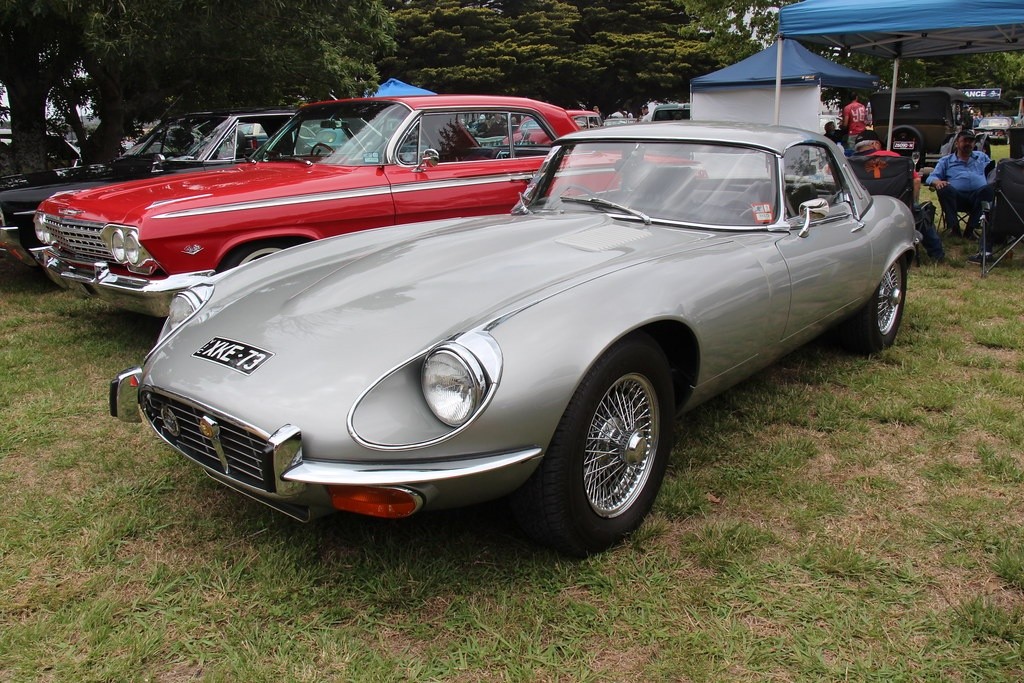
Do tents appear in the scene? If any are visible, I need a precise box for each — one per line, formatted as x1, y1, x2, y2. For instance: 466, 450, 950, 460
363, 78, 438, 99
689, 39, 880, 135
774, 0, 1024, 126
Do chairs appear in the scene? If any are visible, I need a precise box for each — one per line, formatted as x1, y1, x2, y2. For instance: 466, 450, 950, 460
846, 126, 1024, 280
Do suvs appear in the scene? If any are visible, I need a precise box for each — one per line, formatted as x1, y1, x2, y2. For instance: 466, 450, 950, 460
870, 88, 991, 172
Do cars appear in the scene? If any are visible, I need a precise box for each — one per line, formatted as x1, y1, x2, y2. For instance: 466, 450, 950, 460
109, 120, 923, 558
974, 116, 1016, 145
0, 94, 709, 318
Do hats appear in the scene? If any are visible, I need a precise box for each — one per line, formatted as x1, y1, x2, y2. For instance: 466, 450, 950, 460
855, 130, 885, 149
640, 104, 648, 109
593, 106, 598, 110
957, 130, 976, 142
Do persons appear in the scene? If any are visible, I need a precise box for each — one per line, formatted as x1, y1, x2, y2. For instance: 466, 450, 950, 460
640, 104, 652, 122
824, 122, 842, 145
973, 110, 1005, 127
593, 104, 605, 124
925, 130, 992, 241
855, 130, 950, 267
843, 91, 866, 149
967, 158, 1024, 266
473, 113, 508, 136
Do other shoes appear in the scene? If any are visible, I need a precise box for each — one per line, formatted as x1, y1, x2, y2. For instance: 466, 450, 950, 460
969, 252, 993, 262
963, 226, 980, 241
947, 228, 962, 239
